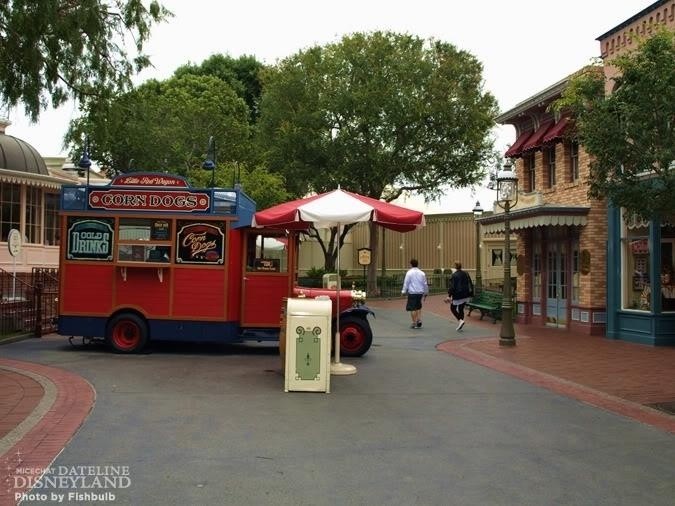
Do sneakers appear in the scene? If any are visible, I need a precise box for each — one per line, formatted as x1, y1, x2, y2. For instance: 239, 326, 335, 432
410, 321, 423, 328
456, 320, 465, 330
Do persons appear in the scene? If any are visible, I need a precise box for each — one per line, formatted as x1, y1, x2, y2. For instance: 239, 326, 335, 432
402, 258, 430, 327
444, 260, 474, 331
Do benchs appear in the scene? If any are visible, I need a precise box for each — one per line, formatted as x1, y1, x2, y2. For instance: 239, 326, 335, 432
466, 290, 504, 324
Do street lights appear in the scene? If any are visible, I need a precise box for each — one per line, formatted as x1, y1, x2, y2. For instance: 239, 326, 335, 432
471, 199, 485, 295
201, 134, 216, 213
492, 157, 521, 349
76, 133, 92, 212
230, 160, 242, 190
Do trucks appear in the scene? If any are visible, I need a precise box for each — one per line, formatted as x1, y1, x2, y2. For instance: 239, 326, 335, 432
56, 174, 376, 361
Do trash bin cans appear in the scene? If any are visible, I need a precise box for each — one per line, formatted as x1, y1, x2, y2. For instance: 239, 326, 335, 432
286, 298, 331, 394
322, 274, 341, 290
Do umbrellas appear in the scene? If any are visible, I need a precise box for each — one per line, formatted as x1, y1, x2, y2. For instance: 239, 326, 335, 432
251, 184, 427, 362
